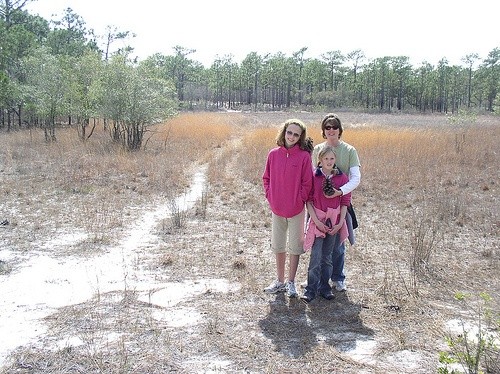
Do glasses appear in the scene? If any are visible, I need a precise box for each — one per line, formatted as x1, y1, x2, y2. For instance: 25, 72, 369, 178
325, 126, 338, 130
286, 130, 301, 137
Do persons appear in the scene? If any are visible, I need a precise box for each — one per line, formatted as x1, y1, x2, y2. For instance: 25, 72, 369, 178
303, 146, 351, 303
262, 118, 314, 298
300, 112, 361, 293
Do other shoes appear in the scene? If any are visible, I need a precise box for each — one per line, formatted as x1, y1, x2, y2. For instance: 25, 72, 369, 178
319, 287, 335, 300
300, 288, 317, 302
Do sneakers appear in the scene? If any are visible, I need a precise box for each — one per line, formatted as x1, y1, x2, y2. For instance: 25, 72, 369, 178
332, 280, 347, 292
285, 279, 298, 298
264, 278, 286, 293
300, 280, 308, 288
